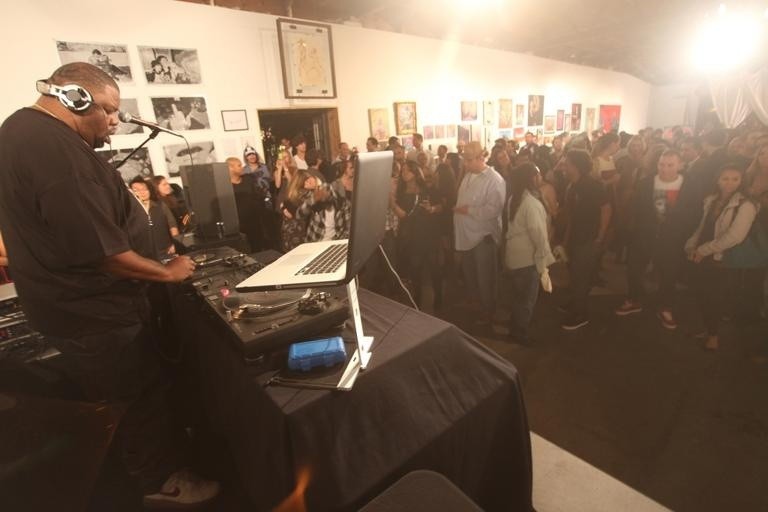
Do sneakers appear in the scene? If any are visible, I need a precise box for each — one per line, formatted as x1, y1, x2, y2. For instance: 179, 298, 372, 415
562, 313, 591, 331
143, 474, 221, 506
657, 307, 676, 329
616, 301, 643, 315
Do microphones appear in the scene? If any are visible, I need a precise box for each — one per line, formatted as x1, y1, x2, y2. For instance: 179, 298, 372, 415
119, 111, 185, 138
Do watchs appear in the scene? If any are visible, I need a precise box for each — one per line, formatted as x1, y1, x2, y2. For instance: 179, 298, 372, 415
430, 205, 434, 214
394, 204, 398, 210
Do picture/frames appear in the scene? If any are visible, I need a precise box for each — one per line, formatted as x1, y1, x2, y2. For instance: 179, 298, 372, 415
368, 107, 390, 143
556, 110, 564, 131
394, 102, 417, 135
276, 17, 337, 99
221, 109, 249, 131
544, 115, 556, 134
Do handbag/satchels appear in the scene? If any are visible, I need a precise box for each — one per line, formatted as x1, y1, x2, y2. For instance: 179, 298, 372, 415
722, 199, 763, 269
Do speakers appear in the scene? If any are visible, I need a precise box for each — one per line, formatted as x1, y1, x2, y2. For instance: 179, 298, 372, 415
178, 162, 241, 239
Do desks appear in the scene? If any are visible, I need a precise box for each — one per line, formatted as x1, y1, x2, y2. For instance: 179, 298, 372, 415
179, 248, 534, 512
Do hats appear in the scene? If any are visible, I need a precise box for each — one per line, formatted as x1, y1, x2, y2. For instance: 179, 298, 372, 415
244, 146, 255, 156
461, 141, 486, 158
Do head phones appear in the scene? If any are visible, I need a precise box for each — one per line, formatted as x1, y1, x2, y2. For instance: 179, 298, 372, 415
33, 79, 92, 113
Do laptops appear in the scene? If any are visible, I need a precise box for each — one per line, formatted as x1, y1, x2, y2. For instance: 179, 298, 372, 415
233, 151, 397, 292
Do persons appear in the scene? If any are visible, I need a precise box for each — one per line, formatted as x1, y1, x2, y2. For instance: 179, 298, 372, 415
366, 134, 465, 309
632, 124, 768, 181
273, 138, 354, 252
506, 162, 554, 348
242, 143, 273, 208
128, 176, 178, 258
150, 56, 178, 85
85, 49, 127, 79
560, 129, 631, 182
619, 137, 638, 264
153, 100, 209, 130
615, 149, 693, 330
685, 162, 758, 353
0, 232, 15, 284
560, 149, 611, 330
147, 177, 178, 223
1, 63, 219, 510
225, 157, 262, 251
453, 142, 506, 312
595, 134, 622, 256
488, 132, 566, 179
